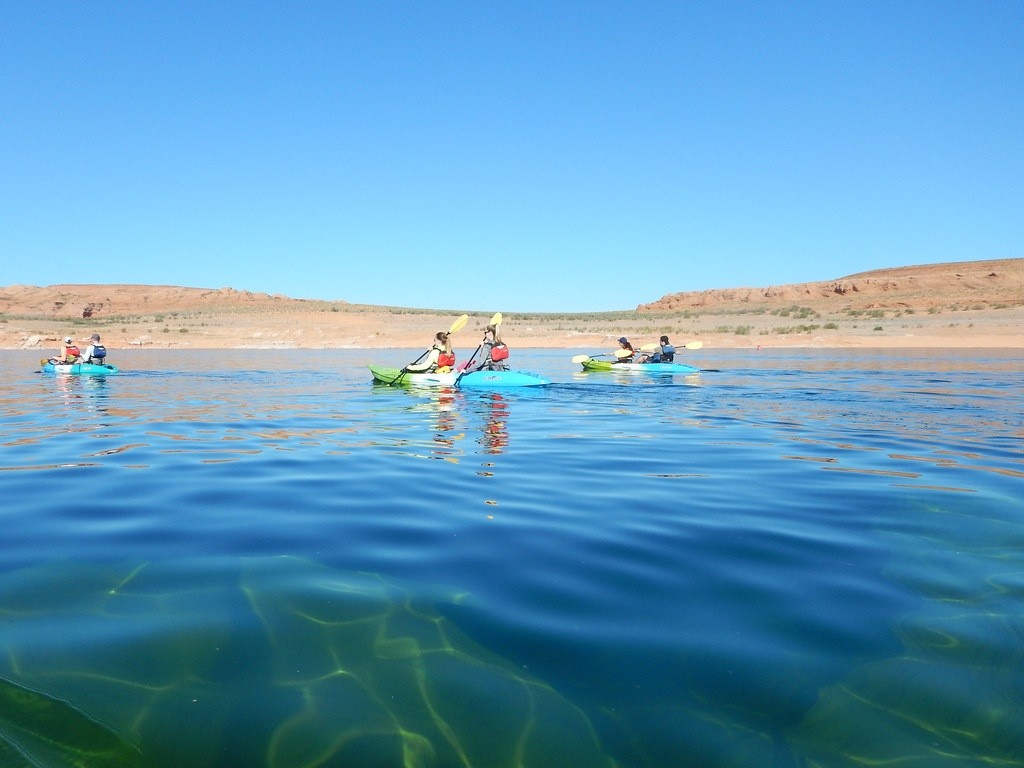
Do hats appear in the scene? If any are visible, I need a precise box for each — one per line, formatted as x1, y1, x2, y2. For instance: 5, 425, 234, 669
91, 334, 100, 339
618, 337, 627, 343
64, 336, 72, 342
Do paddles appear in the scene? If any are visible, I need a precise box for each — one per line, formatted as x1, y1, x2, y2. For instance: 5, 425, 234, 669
451, 312, 502, 386
640, 341, 704, 351
572, 349, 633, 364
40, 354, 61, 366
66, 355, 85, 363
386, 313, 469, 387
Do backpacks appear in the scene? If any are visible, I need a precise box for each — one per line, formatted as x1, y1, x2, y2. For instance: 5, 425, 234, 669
65, 346, 80, 358
433, 348, 455, 367
662, 345, 675, 353
92, 345, 106, 358
485, 342, 508, 360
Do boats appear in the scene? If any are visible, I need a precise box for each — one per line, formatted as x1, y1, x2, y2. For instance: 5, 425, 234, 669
573, 354, 701, 374
367, 364, 552, 388
40, 357, 119, 374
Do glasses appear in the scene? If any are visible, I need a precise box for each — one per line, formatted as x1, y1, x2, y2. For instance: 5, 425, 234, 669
484, 332, 488, 334
434, 339, 435, 341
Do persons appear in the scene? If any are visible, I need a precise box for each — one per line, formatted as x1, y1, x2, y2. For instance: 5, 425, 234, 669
601, 337, 634, 363
459, 325, 504, 373
52, 337, 78, 365
406, 332, 450, 374
82, 334, 106, 366
636, 336, 672, 363
